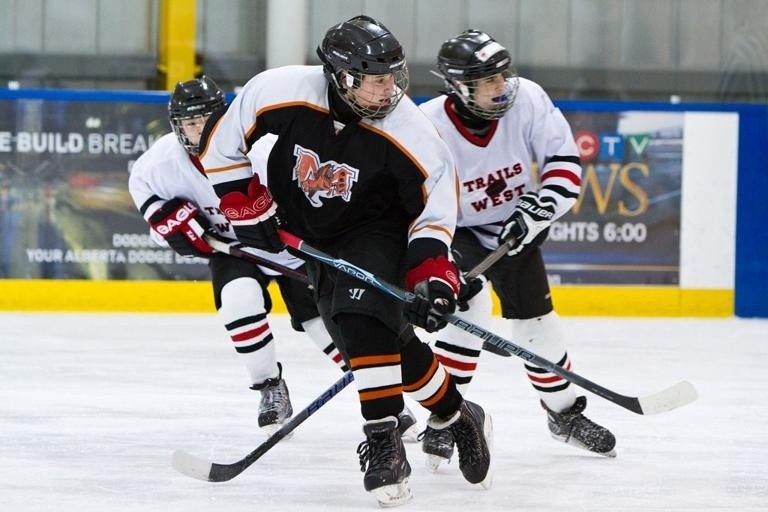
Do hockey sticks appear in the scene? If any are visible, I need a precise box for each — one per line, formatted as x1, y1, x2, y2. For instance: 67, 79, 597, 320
170, 237, 516, 482
276, 230, 707, 416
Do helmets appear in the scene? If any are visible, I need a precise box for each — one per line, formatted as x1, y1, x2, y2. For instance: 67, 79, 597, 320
315, 14, 411, 122
167, 74, 228, 155
429, 23, 521, 120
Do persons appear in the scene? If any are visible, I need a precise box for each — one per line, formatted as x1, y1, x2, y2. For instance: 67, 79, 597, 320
128, 75, 416, 438
416, 29, 616, 460
199, 14, 490, 495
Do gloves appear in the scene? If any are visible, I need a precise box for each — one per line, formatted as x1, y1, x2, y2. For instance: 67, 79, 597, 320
135, 195, 213, 258
217, 173, 290, 254
498, 189, 559, 258
400, 255, 458, 332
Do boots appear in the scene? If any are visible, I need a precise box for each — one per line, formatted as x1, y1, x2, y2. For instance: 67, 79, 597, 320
420, 399, 491, 483
356, 416, 412, 492
538, 395, 617, 452
248, 363, 292, 427
